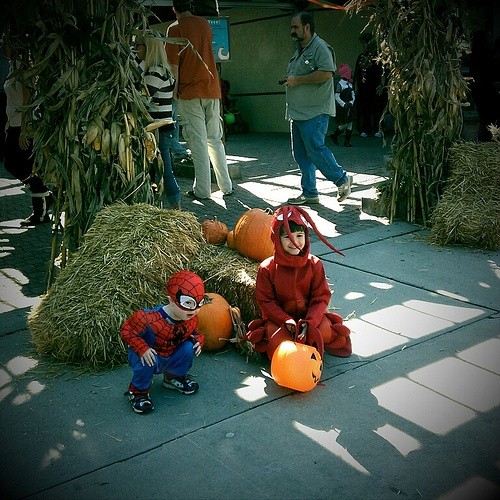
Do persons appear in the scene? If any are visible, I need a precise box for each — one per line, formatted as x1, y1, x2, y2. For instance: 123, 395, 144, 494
357, 31, 385, 138
120, 270, 205, 415
284, 10, 353, 205
255, 204, 352, 358
134, 28, 181, 209
164, 0, 236, 200
330, 63, 356, 148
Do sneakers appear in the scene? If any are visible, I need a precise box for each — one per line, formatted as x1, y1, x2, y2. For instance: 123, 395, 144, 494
374, 132, 381, 137
20, 212, 51, 226
128, 389, 154, 413
161, 372, 199, 394
337, 176, 353, 202
288, 193, 319, 204
360, 132, 368, 137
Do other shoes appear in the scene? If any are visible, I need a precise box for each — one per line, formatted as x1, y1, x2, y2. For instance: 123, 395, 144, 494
223, 187, 234, 195
188, 191, 205, 200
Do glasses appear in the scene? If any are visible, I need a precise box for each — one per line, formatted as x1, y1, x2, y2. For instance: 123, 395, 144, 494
136, 43, 145, 48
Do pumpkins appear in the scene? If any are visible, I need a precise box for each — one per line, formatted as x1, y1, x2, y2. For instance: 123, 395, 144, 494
227, 230, 235, 249
234, 207, 276, 261
194, 293, 232, 352
200, 215, 228, 245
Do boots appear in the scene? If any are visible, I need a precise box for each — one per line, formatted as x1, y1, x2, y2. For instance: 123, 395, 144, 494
330, 126, 344, 145
344, 127, 353, 147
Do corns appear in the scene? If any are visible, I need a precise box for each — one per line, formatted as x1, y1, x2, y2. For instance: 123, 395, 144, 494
146, 134, 155, 161
94, 134, 100, 150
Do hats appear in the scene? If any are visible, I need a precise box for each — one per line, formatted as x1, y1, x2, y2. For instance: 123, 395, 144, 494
338, 63, 353, 82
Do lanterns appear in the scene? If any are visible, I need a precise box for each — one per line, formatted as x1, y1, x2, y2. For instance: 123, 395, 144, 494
270, 339, 324, 393
